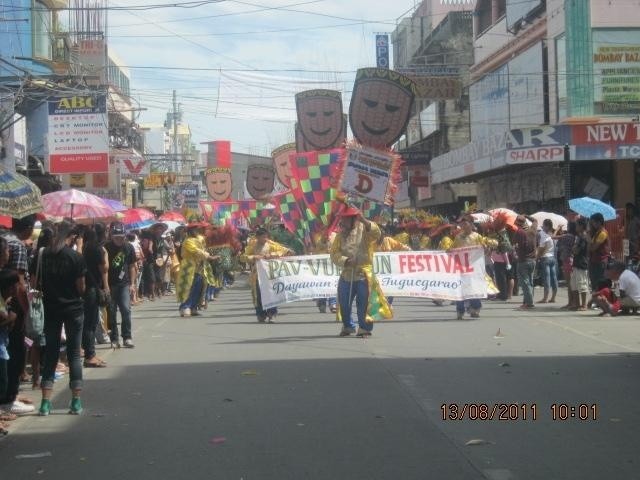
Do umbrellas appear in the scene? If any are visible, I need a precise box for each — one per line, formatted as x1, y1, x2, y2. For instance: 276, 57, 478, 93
568, 196, 617, 221
0, 164, 187, 233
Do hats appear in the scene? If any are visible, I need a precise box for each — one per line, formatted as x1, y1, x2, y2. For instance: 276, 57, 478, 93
342, 208, 359, 217
188, 223, 199, 229
199, 221, 209, 228
111, 221, 126, 237
515, 215, 527, 227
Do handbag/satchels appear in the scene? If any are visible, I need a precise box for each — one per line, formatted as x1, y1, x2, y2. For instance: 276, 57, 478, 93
98, 288, 111, 307
25, 292, 44, 337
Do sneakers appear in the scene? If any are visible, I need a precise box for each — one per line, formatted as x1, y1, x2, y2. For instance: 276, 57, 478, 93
84, 358, 107, 367
70, 396, 82, 414
0, 397, 35, 435
100, 333, 136, 347
40, 398, 51, 416
